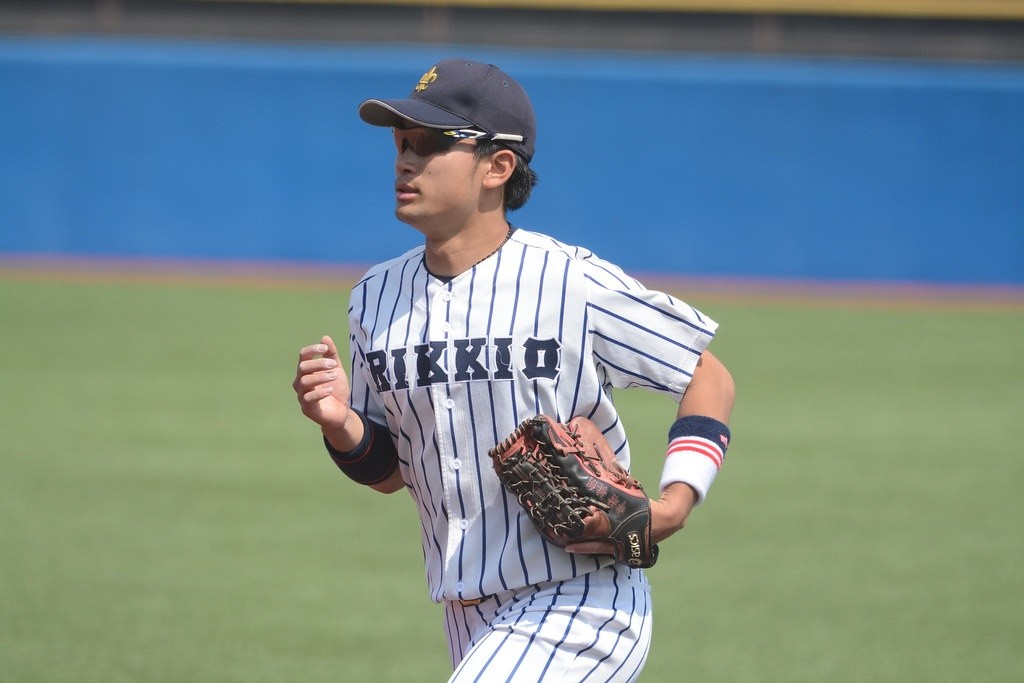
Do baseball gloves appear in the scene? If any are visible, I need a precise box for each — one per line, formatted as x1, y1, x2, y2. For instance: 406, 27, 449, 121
487, 416, 661, 571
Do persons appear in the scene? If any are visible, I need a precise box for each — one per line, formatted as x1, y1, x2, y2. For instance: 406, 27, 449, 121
293, 60, 734, 683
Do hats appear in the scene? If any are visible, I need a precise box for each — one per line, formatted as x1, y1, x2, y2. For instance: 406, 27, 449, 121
358, 58, 536, 162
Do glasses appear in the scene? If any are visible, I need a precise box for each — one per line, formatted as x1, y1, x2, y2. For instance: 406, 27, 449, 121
389, 126, 471, 156
